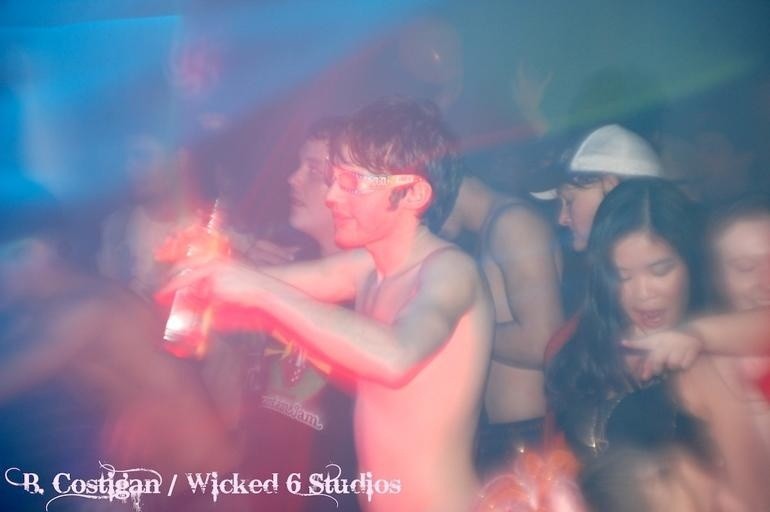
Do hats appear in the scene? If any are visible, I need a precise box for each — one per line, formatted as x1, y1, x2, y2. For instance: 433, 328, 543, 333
527, 122, 667, 202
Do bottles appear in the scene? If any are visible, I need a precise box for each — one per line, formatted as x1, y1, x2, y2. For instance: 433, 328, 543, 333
159, 196, 230, 363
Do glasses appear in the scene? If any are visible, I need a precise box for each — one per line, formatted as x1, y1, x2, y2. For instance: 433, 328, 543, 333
319, 157, 432, 200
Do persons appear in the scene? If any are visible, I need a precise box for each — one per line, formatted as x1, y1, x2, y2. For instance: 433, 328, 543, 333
1, 95, 770, 512
